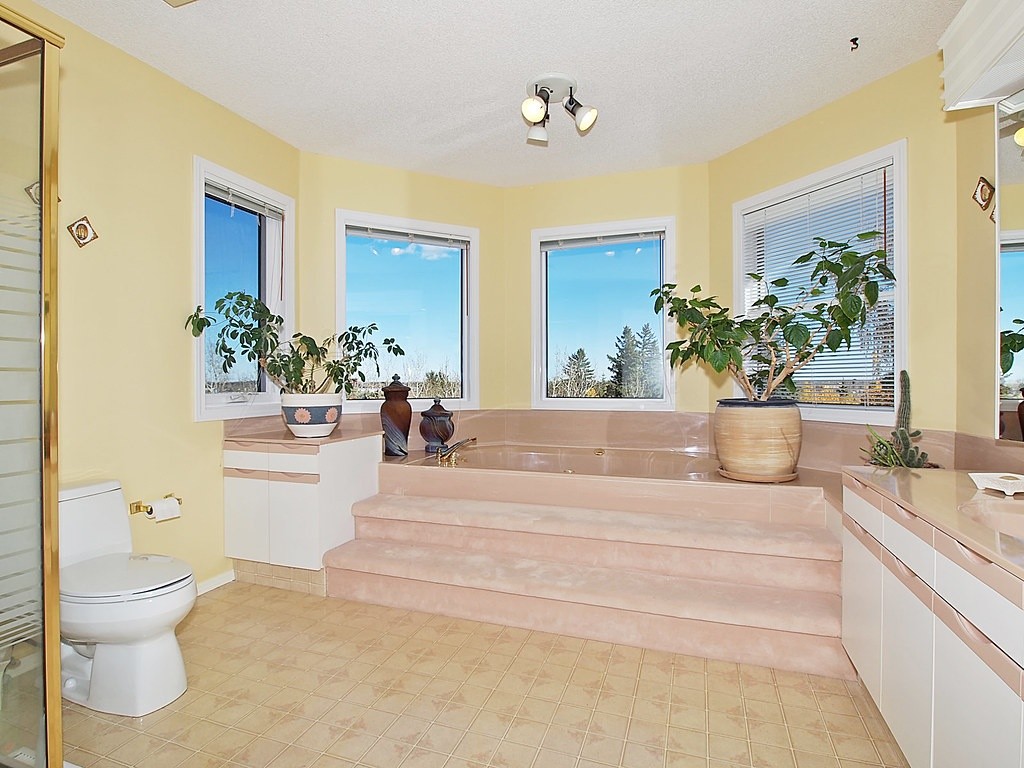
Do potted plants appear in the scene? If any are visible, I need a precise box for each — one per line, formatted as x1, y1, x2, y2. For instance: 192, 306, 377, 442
650, 230, 897, 475
184, 290, 405, 438
1000, 308, 1024, 442
859, 369, 939, 468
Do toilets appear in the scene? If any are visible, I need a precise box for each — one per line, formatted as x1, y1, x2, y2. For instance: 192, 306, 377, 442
58, 480, 198, 718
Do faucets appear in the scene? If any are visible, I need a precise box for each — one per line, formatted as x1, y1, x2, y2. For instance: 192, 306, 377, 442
436, 437, 477, 462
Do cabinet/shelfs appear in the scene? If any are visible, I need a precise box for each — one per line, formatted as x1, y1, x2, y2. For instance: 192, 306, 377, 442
839, 471, 1024, 768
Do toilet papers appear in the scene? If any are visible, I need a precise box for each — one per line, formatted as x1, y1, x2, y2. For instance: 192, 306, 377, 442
144, 497, 181, 523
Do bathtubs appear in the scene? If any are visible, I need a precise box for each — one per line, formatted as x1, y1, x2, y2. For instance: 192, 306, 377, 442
458, 446, 722, 480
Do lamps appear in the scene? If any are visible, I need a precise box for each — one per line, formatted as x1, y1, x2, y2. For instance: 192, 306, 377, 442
527, 112, 549, 143
522, 84, 555, 123
561, 87, 598, 132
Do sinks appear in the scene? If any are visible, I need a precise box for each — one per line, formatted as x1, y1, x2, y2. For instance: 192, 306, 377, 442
956, 496, 1024, 541
